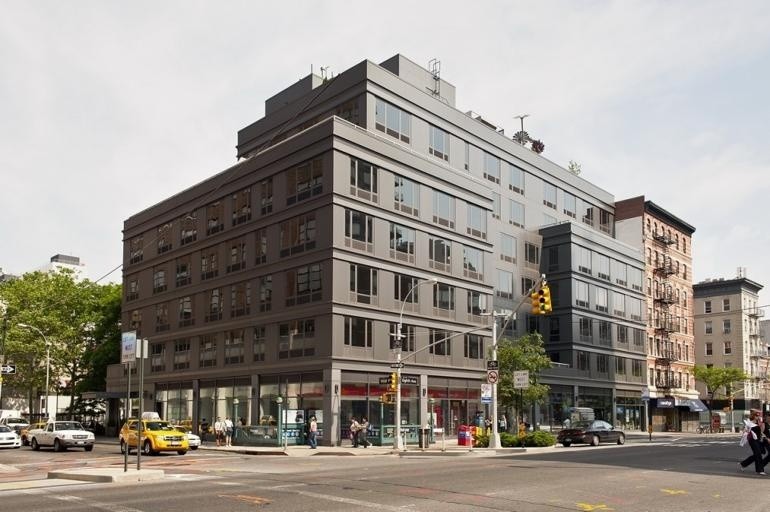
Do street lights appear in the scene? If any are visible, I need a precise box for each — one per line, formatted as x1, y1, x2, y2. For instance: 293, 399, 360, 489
233, 398, 238, 443
17, 323, 50, 421
394, 277, 438, 450
276, 396, 282, 446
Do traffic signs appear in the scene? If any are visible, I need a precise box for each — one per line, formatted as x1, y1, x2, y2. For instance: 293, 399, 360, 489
400, 377, 417, 384
391, 363, 405, 367
122, 331, 137, 361
514, 370, 530, 387
379, 378, 392, 384
1, 366, 16, 374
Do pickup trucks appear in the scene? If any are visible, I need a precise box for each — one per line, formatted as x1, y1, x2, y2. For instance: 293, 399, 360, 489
28, 420, 95, 452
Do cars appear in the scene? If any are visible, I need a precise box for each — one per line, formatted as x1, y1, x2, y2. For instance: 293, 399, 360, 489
173, 425, 201, 450
178, 419, 215, 435
21, 423, 47, 446
0, 417, 30, 429
0, 424, 21, 449
556, 419, 625, 447
259, 414, 277, 426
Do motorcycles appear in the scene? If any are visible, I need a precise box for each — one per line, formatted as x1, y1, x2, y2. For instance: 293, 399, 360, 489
695, 424, 709, 434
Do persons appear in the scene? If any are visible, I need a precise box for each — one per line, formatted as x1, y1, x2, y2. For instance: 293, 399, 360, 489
308, 417, 318, 449
199, 416, 247, 447
350, 417, 362, 447
469, 412, 530, 433
357, 416, 373, 449
761, 411, 770, 467
738, 410, 767, 476
563, 416, 571, 428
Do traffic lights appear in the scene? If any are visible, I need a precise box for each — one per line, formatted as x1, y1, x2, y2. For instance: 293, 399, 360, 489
531, 292, 540, 315
728, 397, 733, 409
538, 286, 551, 313
385, 394, 392, 403
390, 375, 395, 388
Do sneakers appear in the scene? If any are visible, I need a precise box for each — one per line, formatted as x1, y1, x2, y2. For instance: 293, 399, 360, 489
754, 470, 768, 476
736, 462, 745, 474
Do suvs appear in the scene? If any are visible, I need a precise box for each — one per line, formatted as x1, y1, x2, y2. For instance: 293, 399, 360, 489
119, 412, 189, 455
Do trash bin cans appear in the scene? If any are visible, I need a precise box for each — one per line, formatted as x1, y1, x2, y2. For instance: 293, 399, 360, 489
419, 429, 430, 448
